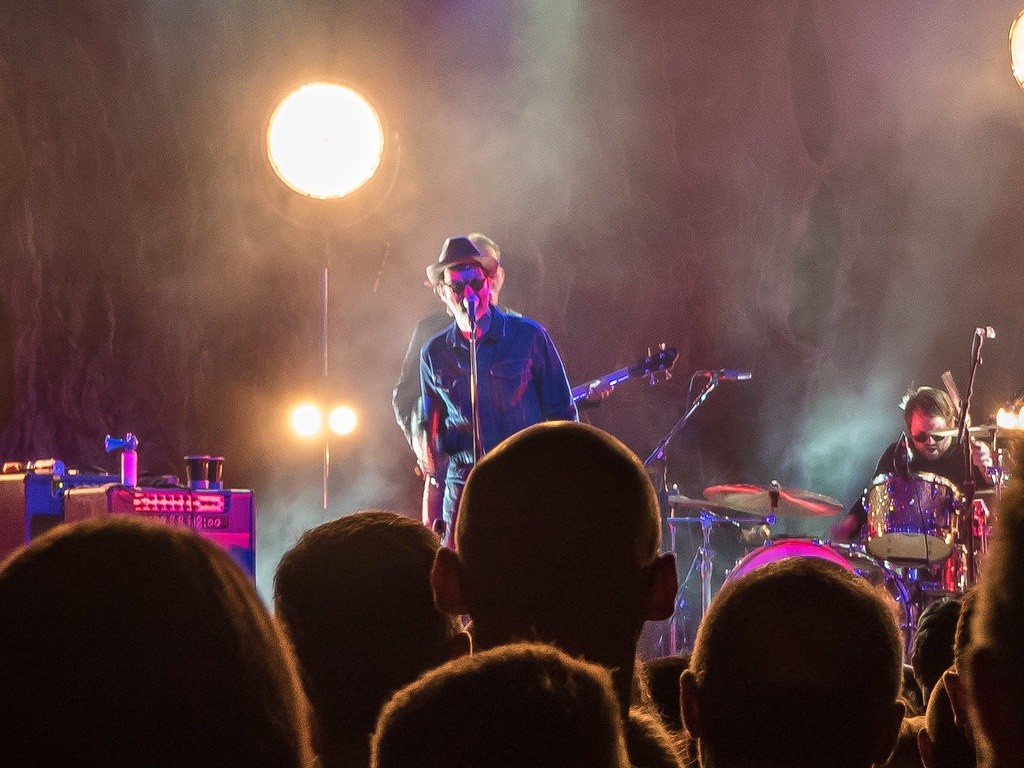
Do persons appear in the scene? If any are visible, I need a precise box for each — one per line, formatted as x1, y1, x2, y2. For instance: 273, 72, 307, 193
391, 232, 614, 549
274, 421, 697, 768
680, 435, 1024, 768
0, 518, 311, 768
833, 384, 994, 560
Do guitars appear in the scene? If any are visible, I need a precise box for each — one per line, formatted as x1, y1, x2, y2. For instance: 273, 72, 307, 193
570, 342, 680, 402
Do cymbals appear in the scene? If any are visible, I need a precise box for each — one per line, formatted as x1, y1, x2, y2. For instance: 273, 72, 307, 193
926, 425, 1001, 438
702, 483, 845, 517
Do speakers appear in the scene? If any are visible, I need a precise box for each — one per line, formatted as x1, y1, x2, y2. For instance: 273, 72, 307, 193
0, 474, 119, 564
62, 482, 256, 597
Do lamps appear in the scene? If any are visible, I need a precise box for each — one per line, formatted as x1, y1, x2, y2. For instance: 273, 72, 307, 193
248, 54, 399, 229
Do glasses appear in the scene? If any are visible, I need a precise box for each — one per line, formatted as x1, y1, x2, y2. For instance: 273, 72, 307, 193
442, 273, 489, 293
907, 429, 946, 442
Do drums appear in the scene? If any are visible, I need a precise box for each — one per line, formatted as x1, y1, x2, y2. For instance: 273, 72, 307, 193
862, 470, 962, 567
838, 547, 915, 655
884, 543, 969, 602
717, 537, 858, 591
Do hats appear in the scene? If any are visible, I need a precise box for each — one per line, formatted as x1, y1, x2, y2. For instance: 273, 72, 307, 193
425, 236, 499, 286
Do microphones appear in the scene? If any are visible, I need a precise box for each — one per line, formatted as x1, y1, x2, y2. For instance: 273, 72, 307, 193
696, 369, 752, 381
893, 432, 912, 462
975, 325, 996, 338
464, 294, 480, 323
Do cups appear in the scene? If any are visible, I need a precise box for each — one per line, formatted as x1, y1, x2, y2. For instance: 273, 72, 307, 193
208, 456, 225, 489
184, 455, 210, 490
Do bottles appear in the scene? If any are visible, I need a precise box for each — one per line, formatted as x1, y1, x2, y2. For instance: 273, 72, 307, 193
121, 433, 138, 487
